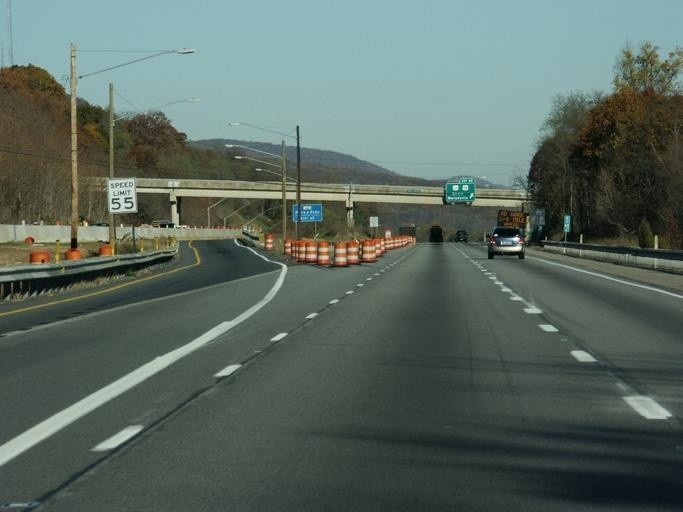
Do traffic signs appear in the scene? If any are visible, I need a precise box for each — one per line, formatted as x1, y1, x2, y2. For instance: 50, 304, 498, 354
445, 183, 475, 202
563, 215, 571, 232
459, 178, 476, 183
291, 204, 323, 222
108, 178, 137, 213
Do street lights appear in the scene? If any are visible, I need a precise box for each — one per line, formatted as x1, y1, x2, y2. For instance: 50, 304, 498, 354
66, 38, 197, 257
222, 118, 304, 254
106, 80, 204, 262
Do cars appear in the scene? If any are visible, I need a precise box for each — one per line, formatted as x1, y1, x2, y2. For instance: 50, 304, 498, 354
89, 216, 189, 229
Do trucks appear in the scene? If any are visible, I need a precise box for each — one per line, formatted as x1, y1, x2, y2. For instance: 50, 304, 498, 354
429, 226, 444, 242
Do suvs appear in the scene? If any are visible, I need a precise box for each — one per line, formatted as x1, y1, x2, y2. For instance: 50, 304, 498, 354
485, 226, 528, 259
454, 229, 469, 243
